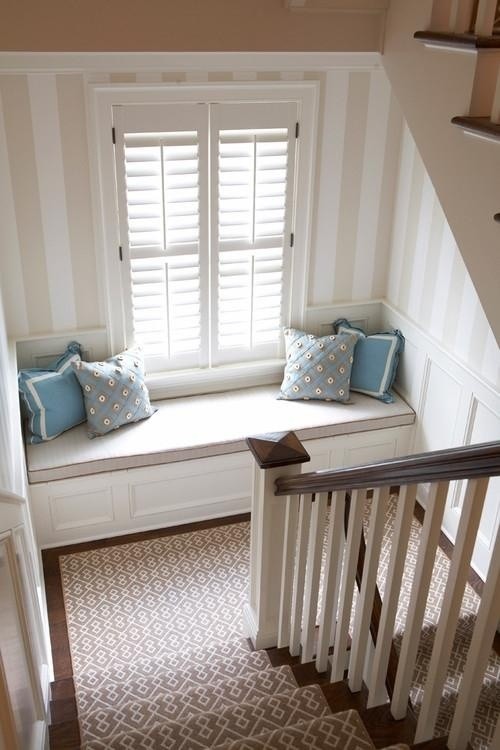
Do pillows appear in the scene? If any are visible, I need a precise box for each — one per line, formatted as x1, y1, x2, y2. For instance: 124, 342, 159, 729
276, 326, 361, 405
332, 318, 407, 405
18, 341, 91, 444
70, 346, 158, 440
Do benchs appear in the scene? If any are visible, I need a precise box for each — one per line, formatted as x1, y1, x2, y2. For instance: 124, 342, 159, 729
24, 374, 418, 550
